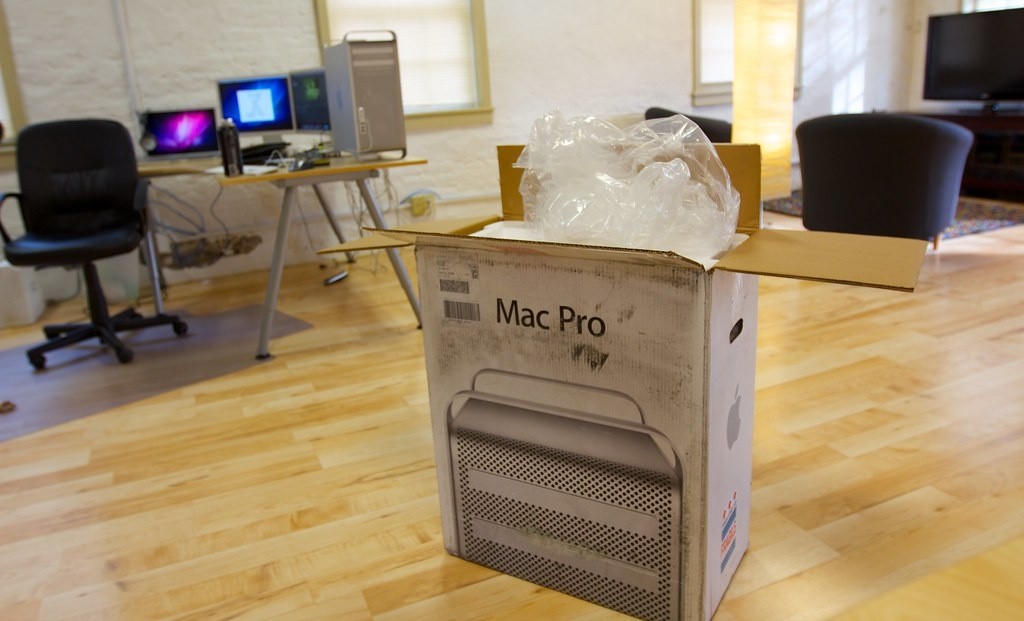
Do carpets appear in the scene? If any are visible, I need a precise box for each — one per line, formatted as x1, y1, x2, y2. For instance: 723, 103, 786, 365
760, 189, 1024, 242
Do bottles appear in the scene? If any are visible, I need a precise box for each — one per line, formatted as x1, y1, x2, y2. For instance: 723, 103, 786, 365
218, 117, 243, 178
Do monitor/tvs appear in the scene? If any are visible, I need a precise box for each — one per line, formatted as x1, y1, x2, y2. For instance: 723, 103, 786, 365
290, 70, 332, 135
143, 108, 222, 160
217, 74, 294, 143
924, 7, 1024, 117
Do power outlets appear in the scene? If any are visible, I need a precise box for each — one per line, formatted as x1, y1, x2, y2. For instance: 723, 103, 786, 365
409, 194, 436, 217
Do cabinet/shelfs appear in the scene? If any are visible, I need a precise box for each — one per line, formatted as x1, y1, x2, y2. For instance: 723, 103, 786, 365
873, 110, 1024, 202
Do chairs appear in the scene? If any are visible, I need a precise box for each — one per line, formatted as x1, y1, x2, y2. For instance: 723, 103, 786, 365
0, 119, 187, 368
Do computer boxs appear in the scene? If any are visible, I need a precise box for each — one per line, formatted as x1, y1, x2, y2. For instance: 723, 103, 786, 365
324, 30, 407, 164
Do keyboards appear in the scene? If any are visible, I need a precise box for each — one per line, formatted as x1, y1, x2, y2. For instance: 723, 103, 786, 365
204, 163, 279, 176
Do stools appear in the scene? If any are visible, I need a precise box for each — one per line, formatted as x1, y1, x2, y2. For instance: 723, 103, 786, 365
795, 113, 974, 250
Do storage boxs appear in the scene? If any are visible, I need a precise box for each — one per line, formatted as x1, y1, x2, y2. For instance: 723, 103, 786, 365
316, 145, 929, 621
0, 260, 46, 330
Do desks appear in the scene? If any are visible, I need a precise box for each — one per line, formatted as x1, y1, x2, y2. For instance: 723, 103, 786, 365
137, 149, 427, 358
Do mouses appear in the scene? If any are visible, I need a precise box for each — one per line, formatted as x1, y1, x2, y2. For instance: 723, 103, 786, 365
288, 157, 314, 172
